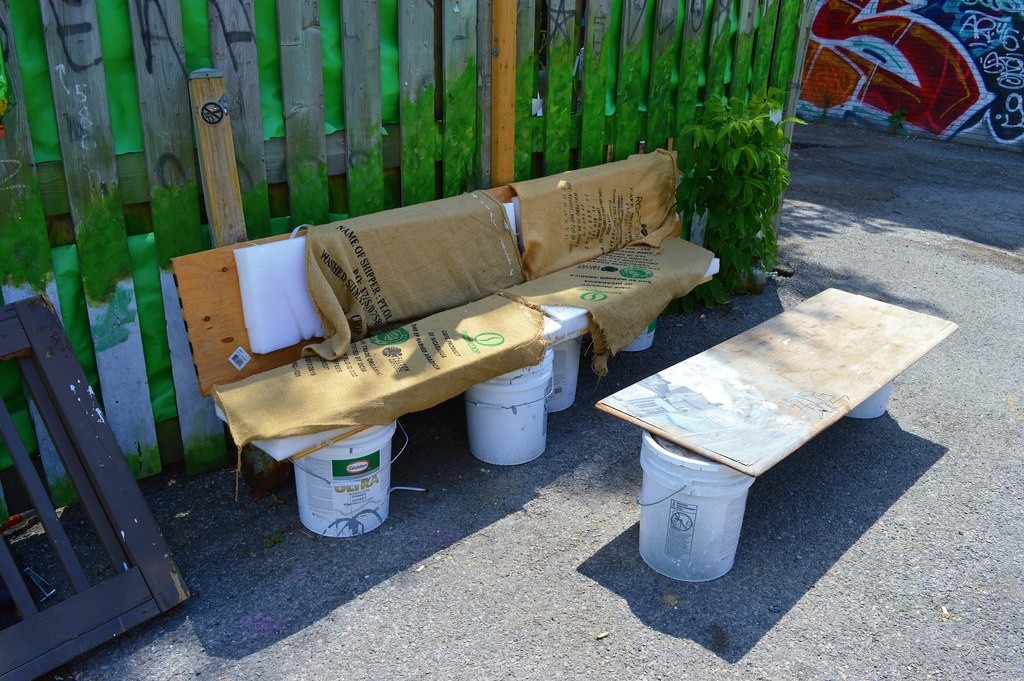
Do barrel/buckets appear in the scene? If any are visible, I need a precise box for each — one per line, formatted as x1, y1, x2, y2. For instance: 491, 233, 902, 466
619, 316, 656, 352
844, 379, 894, 419
548, 338, 582, 413
291, 420, 395, 539
465, 350, 553, 465
640, 429, 755, 582
